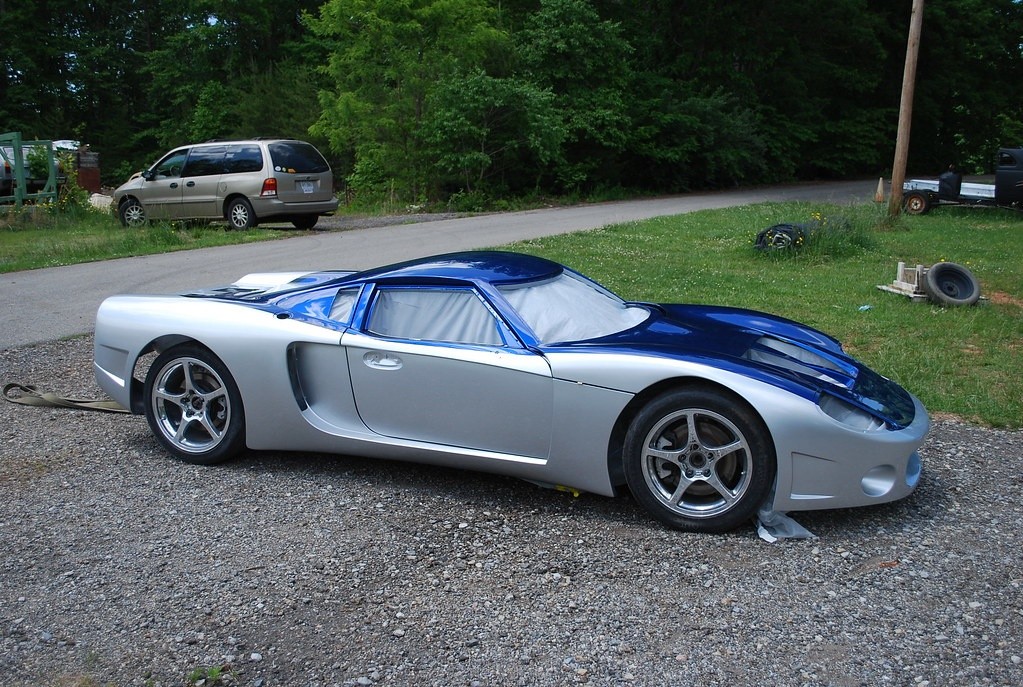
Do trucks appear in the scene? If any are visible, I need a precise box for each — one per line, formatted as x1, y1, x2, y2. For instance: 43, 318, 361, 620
900, 148, 1023, 215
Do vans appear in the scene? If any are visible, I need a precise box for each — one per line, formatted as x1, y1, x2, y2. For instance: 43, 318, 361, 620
110, 139, 341, 229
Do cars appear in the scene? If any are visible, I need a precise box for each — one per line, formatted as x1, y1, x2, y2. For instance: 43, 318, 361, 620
0, 145, 67, 204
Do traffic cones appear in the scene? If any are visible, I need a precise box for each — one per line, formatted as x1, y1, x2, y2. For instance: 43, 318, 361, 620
872, 176, 888, 204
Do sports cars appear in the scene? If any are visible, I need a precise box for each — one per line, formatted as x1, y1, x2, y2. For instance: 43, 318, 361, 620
89, 247, 934, 537
